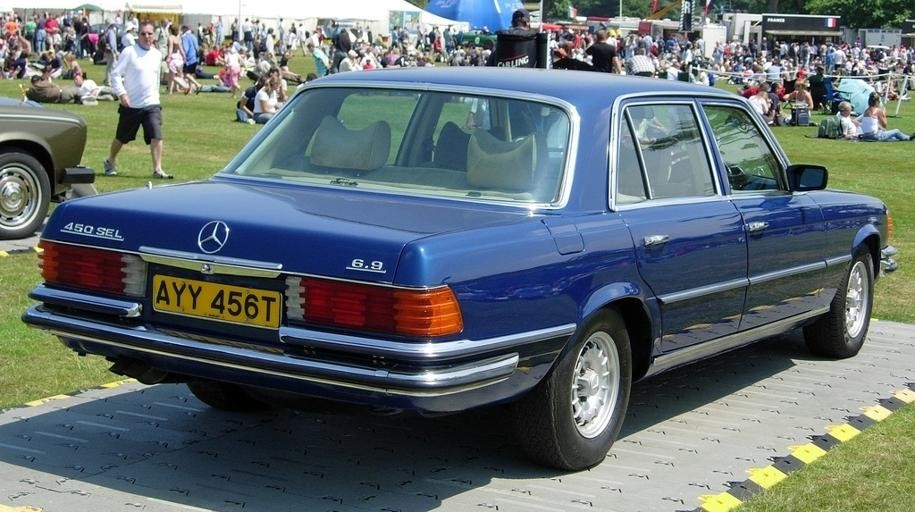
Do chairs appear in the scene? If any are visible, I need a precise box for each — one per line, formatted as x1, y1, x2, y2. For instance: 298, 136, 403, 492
820, 79, 853, 115
434, 118, 506, 172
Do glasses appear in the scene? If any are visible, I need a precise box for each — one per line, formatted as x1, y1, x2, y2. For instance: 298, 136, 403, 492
141, 31, 153, 36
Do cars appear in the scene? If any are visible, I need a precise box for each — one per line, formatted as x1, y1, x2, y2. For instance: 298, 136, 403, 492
0, 97, 94, 239
21, 66, 898, 471
89, 23, 129, 51
452, 30, 498, 51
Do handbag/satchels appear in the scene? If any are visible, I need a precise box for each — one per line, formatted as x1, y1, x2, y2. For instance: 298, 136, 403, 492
819, 116, 842, 138
792, 108, 808, 125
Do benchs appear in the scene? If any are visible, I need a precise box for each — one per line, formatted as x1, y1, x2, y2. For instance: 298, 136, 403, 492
275, 114, 559, 206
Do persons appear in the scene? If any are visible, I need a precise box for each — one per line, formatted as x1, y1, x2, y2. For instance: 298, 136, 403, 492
306, 7, 714, 128
708, 37, 914, 142
0, 7, 309, 180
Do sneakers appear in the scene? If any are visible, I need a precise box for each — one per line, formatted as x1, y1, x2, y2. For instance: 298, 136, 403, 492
104, 158, 117, 174
153, 170, 174, 179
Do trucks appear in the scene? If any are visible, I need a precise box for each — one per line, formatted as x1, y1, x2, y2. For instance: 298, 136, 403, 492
527, 11, 734, 58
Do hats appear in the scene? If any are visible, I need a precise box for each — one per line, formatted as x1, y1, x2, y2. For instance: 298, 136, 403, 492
512, 8, 530, 19
